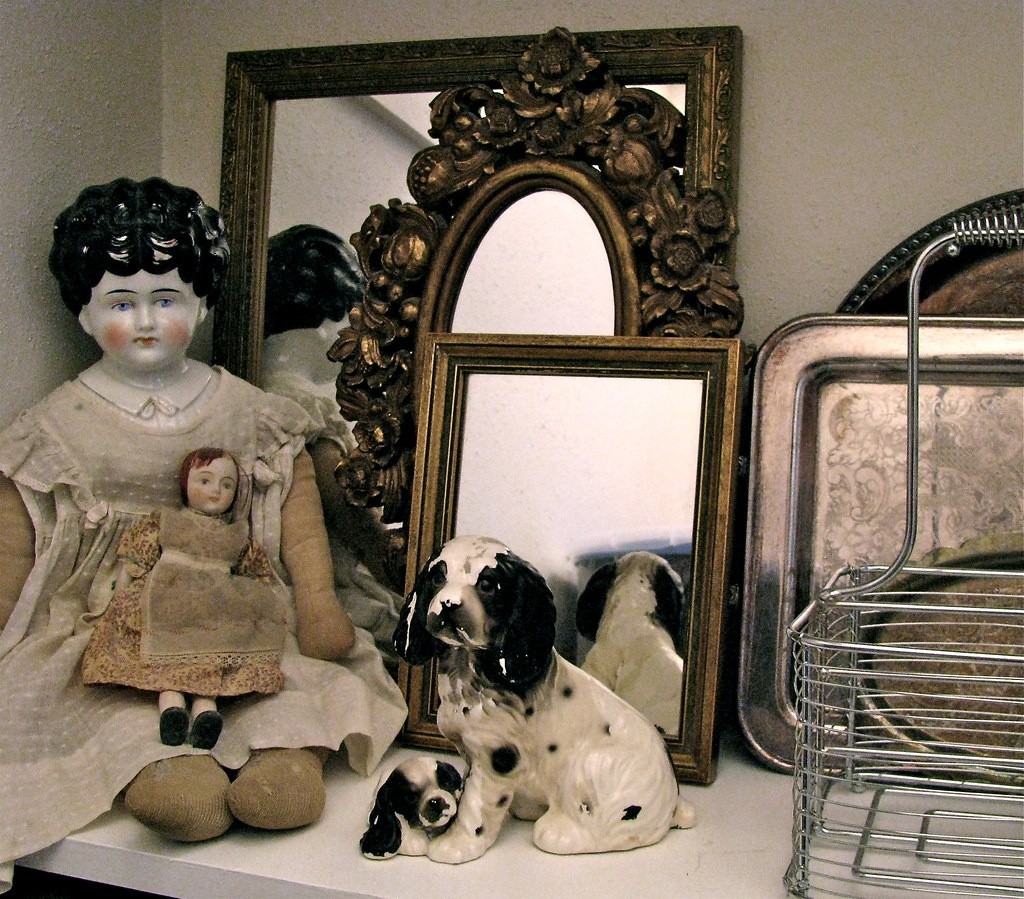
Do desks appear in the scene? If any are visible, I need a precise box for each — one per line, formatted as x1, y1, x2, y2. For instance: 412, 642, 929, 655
13, 737, 1024, 899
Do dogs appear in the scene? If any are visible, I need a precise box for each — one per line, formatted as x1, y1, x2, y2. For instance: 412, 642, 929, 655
358, 533, 697, 866
574, 550, 686, 740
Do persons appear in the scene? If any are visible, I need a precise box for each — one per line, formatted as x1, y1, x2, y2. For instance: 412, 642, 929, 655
80, 444, 290, 751
1, 178, 411, 897
263, 221, 407, 659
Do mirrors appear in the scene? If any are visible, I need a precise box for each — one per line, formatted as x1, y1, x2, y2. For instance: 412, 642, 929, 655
327, 22, 750, 605
207, 22, 747, 686
397, 330, 747, 788
